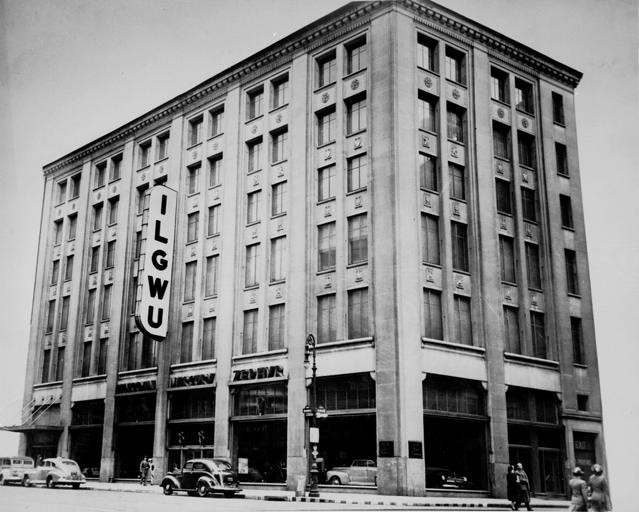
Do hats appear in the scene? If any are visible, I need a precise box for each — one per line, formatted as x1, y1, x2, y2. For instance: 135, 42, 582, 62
572, 463, 603, 475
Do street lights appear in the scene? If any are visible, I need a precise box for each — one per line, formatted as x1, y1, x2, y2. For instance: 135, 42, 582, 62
304, 334, 319, 497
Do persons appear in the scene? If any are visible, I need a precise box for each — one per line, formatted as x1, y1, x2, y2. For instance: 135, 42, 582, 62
36, 455, 45, 467
140, 456, 151, 487
589, 464, 613, 512
507, 465, 518, 510
513, 463, 534, 511
148, 458, 155, 486
566, 467, 591, 512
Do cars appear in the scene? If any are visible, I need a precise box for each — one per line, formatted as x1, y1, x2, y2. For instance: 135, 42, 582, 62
0, 455, 87, 489
426, 468, 468, 489
159, 458, 244, 497
327, 460, 377, 486
